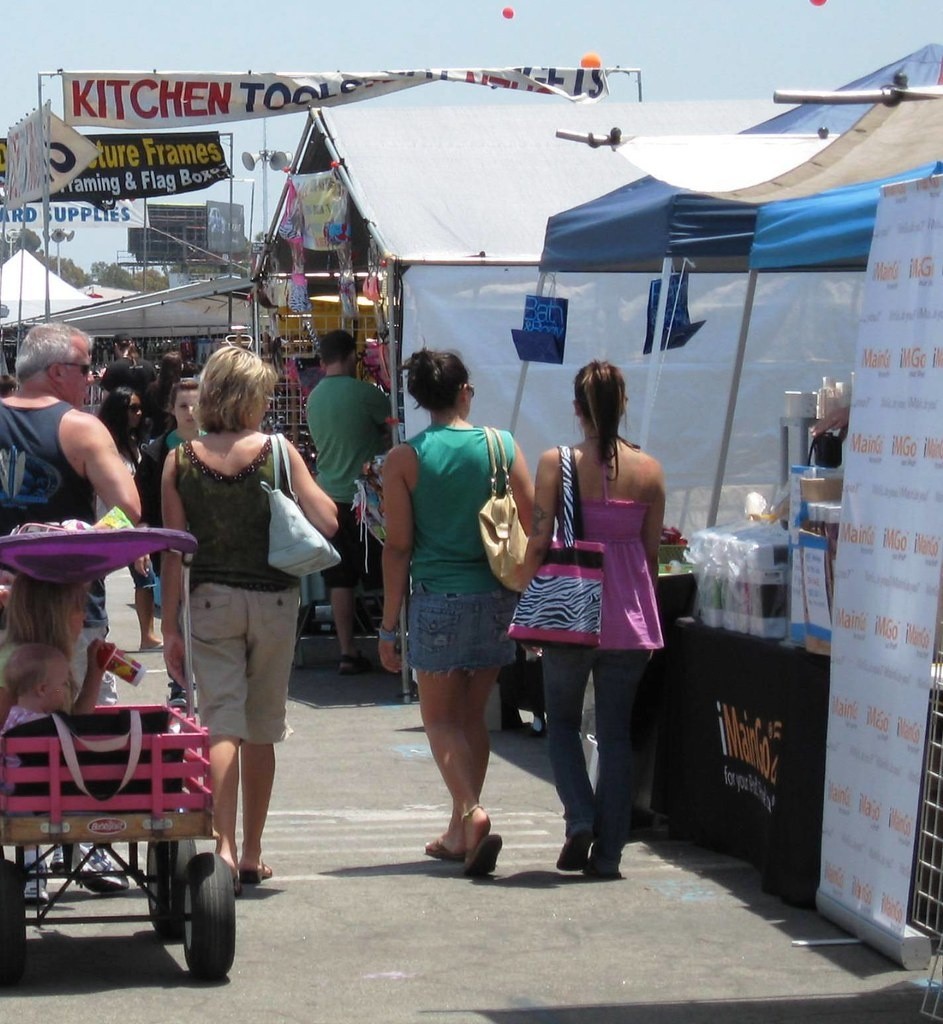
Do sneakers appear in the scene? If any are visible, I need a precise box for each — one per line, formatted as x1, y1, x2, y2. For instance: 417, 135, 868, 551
50, 842, 130, 893
23, 849, 49, 905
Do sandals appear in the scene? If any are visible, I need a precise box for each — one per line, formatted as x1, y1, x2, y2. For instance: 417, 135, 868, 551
338, 650, 372, 675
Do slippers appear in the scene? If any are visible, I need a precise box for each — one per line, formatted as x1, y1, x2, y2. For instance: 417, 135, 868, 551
139, 642, 164, 652
233, 876, 242, 897
462, 834, 502, 877
426, 837, 465, 862
239, 859, 272, 884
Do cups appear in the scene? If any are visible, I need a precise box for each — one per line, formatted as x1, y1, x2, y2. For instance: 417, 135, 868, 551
664, 563, 672, 572
785, 390, 817, 417
814, 436, 842, 467
819, 377, 848, 419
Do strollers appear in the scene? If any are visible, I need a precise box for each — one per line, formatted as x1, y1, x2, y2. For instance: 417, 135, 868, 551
1, 517, 237, 983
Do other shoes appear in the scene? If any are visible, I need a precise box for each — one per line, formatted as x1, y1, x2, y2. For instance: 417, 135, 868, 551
557, 829, 593, 871
582, 859, 622, 880
168, 680, 196, 707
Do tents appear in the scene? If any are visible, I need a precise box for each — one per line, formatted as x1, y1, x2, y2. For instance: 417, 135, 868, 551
511, 44, 942, 527
253, 99, 810, 704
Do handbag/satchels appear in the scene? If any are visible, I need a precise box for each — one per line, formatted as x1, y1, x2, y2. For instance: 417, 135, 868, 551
478, 426, 530, 593
3, 709, 184, 816
507, 445, 606, 650
643, 262, 706, 354
511, 271, 568, 364
260, 433, 342, 578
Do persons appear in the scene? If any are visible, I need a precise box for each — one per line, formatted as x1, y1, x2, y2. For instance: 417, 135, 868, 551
521, 360, 665, 876
0, 575, 107, 817
161, 346, 338, 897
377, 351, 537, 875
810, 406, 851, 436
1, 322, 141, 902
99, 333, 207, 652
306, 330, 391, 674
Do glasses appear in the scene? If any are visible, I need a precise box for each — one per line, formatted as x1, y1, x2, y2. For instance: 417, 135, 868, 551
459, 383, 475, 398
128, 404, 144, 411
46, 362, 91, 377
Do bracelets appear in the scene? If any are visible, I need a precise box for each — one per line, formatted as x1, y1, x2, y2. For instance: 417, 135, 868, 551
378, 627, 396, 640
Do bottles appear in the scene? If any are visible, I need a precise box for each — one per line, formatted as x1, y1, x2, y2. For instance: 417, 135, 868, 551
788, 464, 812, 531
97, 643, 146, 687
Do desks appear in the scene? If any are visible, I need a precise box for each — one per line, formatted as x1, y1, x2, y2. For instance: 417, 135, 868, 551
653, 612, 831, 911
